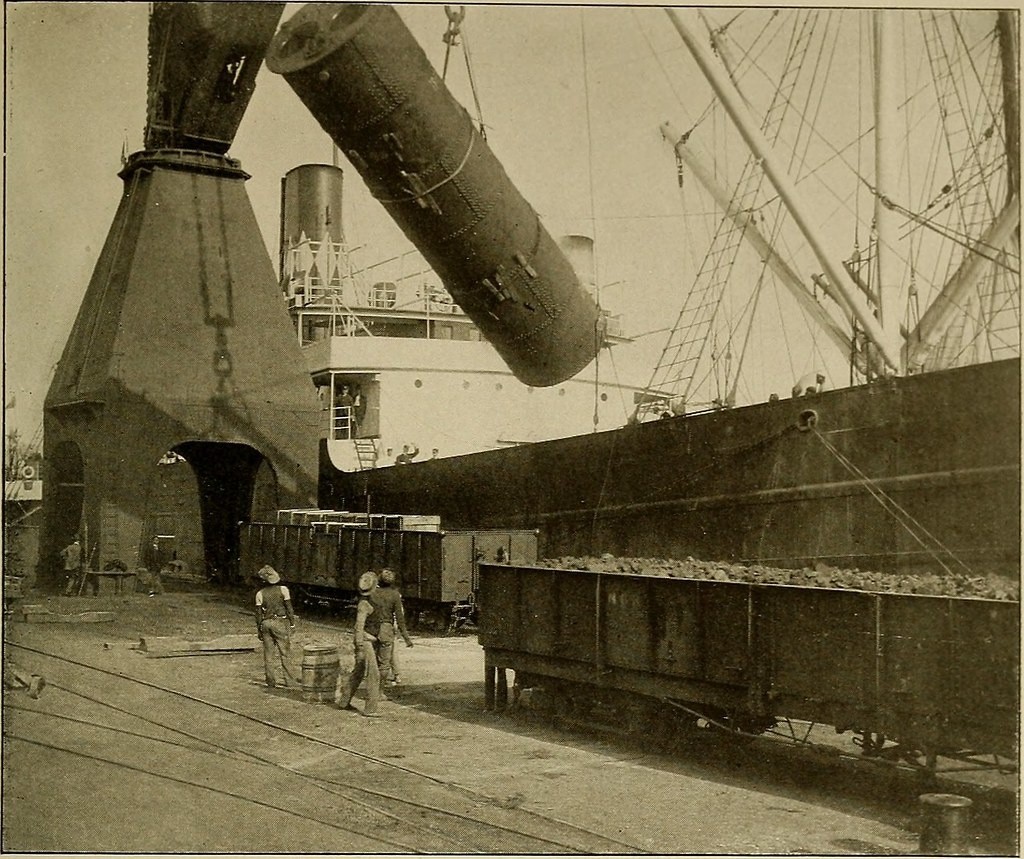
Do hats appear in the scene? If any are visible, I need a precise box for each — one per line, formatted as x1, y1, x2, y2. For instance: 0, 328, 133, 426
357, 571, 378, 596
380, 569, 395, 585
258, 565, 280, 584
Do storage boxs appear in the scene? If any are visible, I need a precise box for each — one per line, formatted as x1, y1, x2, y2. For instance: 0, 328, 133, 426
277, 508, 440, 533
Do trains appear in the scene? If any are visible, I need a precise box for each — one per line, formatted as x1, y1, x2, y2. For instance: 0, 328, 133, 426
233, 508, 539, 637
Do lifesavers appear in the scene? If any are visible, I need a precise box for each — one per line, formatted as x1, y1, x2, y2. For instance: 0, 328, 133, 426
22, 466, 35, 479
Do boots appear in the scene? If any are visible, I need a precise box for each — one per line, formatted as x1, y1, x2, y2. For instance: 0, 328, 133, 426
378, 669, 392, 702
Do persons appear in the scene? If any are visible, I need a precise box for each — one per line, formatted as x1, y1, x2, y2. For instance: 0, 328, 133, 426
59, 533, 86, 599
376, 443, 441, 468
652, 378, 819, 421
255, 562, 302, 690
144, 534, 169, 597
338, 571, 385, 721
333, 383, 367, 439
376, 568, 414, 690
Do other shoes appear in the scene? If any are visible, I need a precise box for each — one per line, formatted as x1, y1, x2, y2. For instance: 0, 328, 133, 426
362, 710, 384, 717
394, 673, 401, 683
339, 704, 357, 710
287, 678, 302, 687
